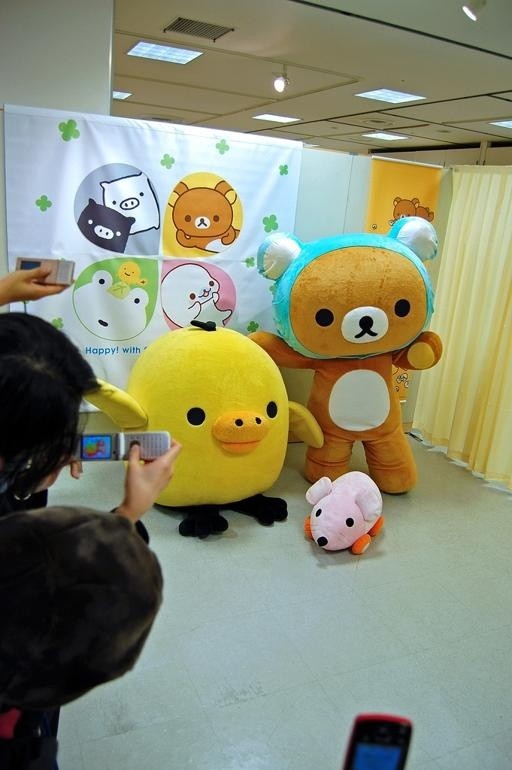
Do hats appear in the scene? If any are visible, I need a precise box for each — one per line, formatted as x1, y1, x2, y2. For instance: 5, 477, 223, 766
0, 506, 162, 705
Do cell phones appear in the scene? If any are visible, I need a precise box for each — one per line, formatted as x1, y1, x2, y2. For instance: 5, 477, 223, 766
343, 711, 412, 770
74, 430, 170, 462
14, 256, 76, 286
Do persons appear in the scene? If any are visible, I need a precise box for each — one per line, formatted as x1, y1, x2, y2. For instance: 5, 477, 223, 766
0, 265, 75, 306
0, 505, 163, 770
0, 312, 182, 543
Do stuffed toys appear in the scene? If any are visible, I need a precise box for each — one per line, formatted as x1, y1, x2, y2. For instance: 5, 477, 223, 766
305, 470, 385, 555
83, 321, 324, 538
246, 216, 443, 495
